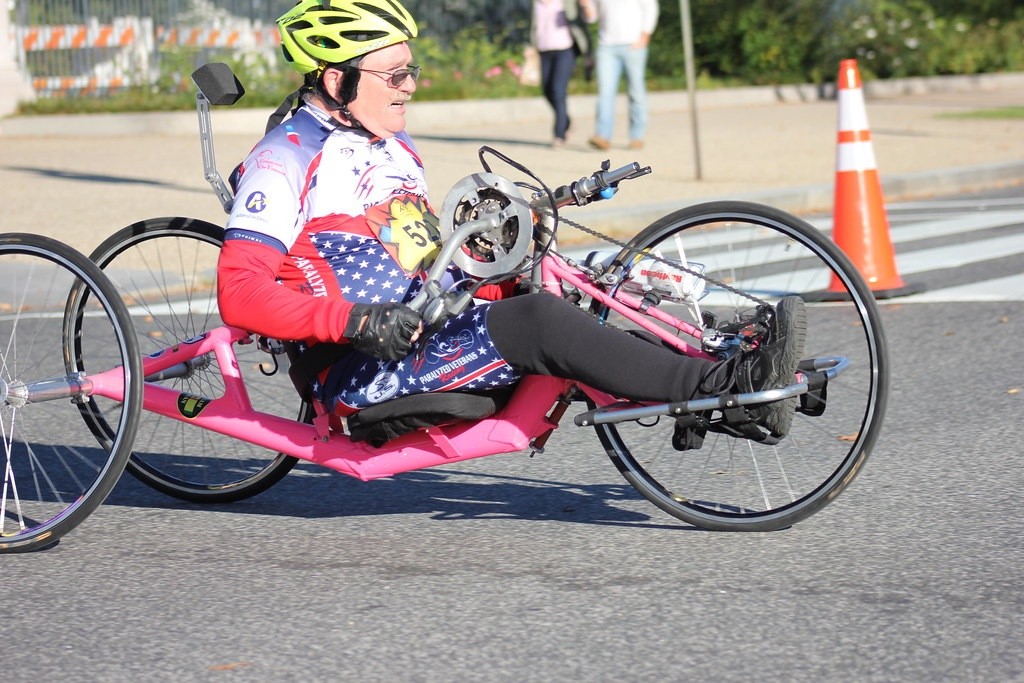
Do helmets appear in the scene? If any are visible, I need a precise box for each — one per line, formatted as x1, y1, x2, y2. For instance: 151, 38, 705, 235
276, 0, 419, 78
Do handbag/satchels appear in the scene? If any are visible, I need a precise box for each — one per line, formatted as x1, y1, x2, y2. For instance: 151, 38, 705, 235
517, 48, 543, 87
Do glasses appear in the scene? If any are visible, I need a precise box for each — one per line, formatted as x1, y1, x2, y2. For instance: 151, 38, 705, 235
359, 64, 423, 86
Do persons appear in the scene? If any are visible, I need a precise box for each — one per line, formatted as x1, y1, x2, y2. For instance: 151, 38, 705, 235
579, 0, 660, 152
528, 0, 591, 148
214, 0, 809, 445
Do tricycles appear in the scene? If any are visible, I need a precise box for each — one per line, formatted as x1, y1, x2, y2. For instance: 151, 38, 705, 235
0, 61, 889, 552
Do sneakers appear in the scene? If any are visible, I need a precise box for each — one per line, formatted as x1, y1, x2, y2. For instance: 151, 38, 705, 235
732, 295, 807, 436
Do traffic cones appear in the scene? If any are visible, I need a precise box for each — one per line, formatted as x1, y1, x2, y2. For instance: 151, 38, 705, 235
798, 58, 927, 302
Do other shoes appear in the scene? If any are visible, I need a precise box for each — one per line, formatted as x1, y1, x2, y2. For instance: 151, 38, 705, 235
588, 137, 608, 149
550, 138, 563, 149
627, 139, 644, 149
